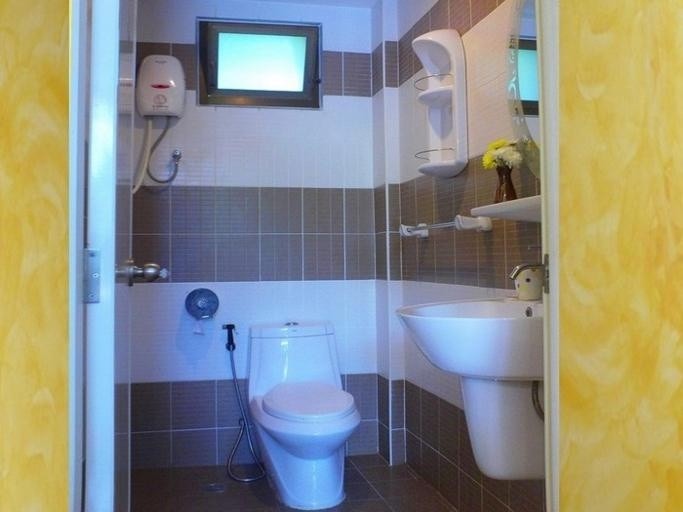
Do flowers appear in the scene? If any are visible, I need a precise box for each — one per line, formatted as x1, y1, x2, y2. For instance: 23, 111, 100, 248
480, 137, 524, 172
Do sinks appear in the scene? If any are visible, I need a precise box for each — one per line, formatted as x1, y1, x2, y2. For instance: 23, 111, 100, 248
395, 296, 544, 379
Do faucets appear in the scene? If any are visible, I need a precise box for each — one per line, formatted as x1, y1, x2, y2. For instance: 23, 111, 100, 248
509, 262, 547, 279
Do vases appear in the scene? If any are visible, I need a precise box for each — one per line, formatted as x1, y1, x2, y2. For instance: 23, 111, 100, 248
493, 165, 517, 204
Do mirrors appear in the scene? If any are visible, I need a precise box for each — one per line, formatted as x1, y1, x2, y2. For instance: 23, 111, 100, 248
503, 0, 539, 183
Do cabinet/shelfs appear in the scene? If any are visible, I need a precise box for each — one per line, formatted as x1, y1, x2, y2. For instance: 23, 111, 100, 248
410, 27, 470, 180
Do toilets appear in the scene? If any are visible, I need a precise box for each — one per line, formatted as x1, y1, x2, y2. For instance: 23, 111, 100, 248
244, 321, 362, 507
